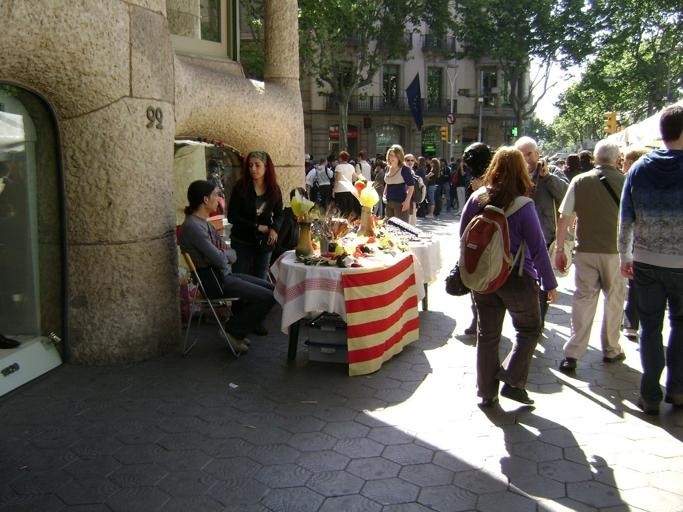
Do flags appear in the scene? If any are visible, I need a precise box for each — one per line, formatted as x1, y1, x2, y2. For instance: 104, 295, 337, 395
405, 72, 424, 131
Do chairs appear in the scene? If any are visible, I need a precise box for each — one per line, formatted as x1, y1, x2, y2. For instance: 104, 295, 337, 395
179, 243, 243, 358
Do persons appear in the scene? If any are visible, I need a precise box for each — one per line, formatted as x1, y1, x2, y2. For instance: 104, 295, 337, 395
457, 147, 559, 411
0, 333, 21, 349
0, 161, 25, 332
458, 141, 493, 337
617, 104, 683, 417
511, 135, 569, 328
382, 143, 415, 224
552, 136, 627, 376
538, 151, 653, 342
225, 150, 284, 335
175, 179, 276, 353
272, 185, 310, 265
306, 150, 473, 219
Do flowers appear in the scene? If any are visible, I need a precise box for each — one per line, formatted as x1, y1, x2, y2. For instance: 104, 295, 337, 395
291, 183, 315, 223
339, 173, 383, 207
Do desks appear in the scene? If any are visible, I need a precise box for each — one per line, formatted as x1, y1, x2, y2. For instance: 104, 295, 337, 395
277, 220, 440, 372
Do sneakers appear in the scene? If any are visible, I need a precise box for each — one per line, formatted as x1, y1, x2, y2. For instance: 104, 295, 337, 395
453, 211, 462, 216
252, 322, 268, 335
424, 215, 434, 219
482, 394, 498, 407
665, 391, 682, 406
464, 328, 475, 334
603, 349, 625, 362
501, 382, 534, 404
637, 395, 659, 415
560, 356, 576, 370
623, 327, 637, 337
218, 325, 250, 353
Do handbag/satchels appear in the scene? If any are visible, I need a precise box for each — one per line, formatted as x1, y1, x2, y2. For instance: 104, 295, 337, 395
459, 185, 535, 295
191, 265, 225, 299
412, 175, 427, 203
548, 239, 573, 277
446, 264, 471, 295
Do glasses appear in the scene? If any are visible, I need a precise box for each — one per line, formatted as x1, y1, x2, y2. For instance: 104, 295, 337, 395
407, 159, 414, 162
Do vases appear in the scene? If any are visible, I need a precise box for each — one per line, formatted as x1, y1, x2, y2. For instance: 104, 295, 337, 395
357, 206, 375, 236
